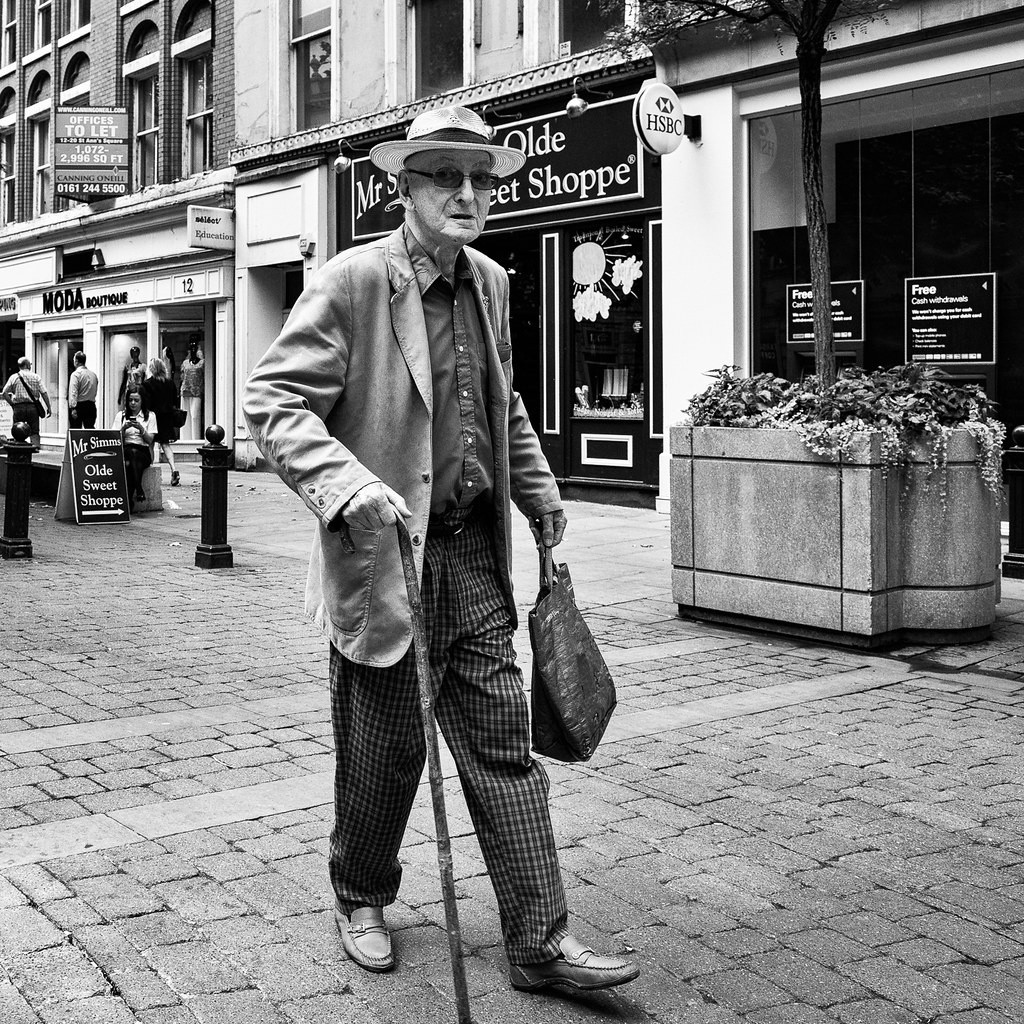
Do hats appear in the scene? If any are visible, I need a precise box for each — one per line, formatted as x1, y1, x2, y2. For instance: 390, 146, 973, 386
370, 107, 528, 176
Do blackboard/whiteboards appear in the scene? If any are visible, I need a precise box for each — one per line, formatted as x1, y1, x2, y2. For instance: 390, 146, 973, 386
54, 427, 130, 526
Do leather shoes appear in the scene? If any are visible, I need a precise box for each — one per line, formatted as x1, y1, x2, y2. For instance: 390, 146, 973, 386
335, 907, 393, 970
511, 934, 640, 990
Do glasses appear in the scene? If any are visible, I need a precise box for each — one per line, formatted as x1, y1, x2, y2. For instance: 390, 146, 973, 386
403, 168, 502, 191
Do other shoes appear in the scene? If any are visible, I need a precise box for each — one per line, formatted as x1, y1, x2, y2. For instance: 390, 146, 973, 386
171, 471, 180, 486
136, 490, 145, 502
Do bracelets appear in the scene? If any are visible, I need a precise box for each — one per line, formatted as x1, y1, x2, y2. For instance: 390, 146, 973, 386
142, 429, 145, 436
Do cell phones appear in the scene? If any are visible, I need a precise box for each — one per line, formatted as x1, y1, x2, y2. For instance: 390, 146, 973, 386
130, 417, 136, 422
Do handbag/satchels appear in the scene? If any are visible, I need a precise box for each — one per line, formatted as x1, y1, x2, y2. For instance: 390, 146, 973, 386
528, 547, 616, 762
173, 409, 187, 428
36, 400, 45, 417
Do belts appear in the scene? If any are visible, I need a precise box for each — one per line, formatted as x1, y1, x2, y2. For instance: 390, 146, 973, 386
428, 515, 477, 538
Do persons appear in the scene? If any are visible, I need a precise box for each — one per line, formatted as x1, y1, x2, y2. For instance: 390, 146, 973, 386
112, 384, 158, 514
242, 107, 640, 991
118, 347, 146, 405
180, 342, 205, 440
141, 358, 181, 486
162, 346, 175, 380
2, 357, 52, 446
69, 351, 98, 429
